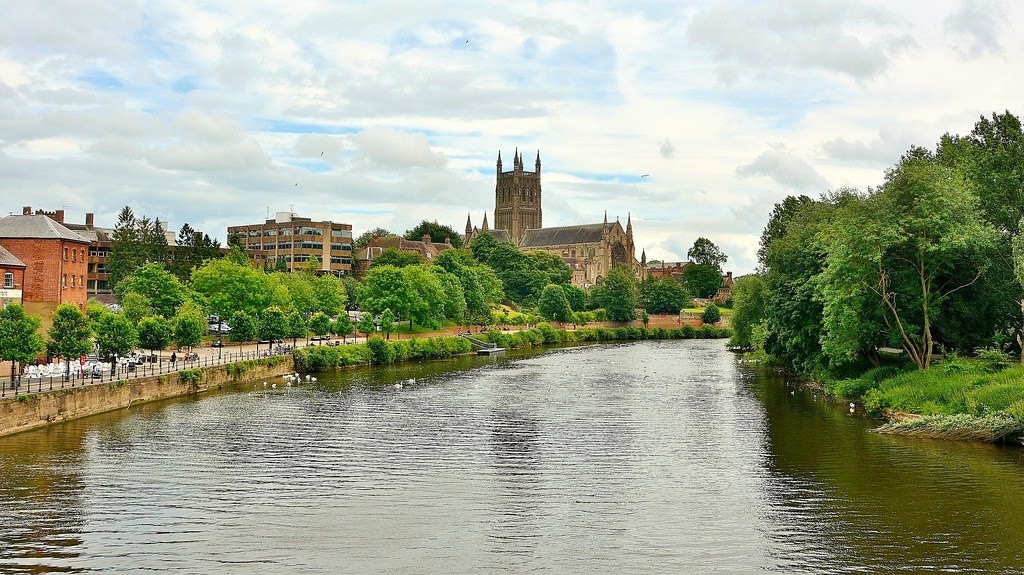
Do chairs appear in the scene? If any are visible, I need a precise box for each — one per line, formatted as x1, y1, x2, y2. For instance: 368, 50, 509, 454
23, 355, 142, 379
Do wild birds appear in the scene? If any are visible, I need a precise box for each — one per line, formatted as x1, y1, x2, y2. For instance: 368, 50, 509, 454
262, 381, 268, 387
271, 383, 277, 388
393, 377, 416, 390
849, 402, 856, 414
737, 358, 744, 364
791, 391, 794, 395
283, 371, 318, 387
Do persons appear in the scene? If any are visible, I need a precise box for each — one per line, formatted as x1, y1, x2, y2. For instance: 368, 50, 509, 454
170, 352, 176, 362
458, 326, 486, 336
185, 352, 189, 360
